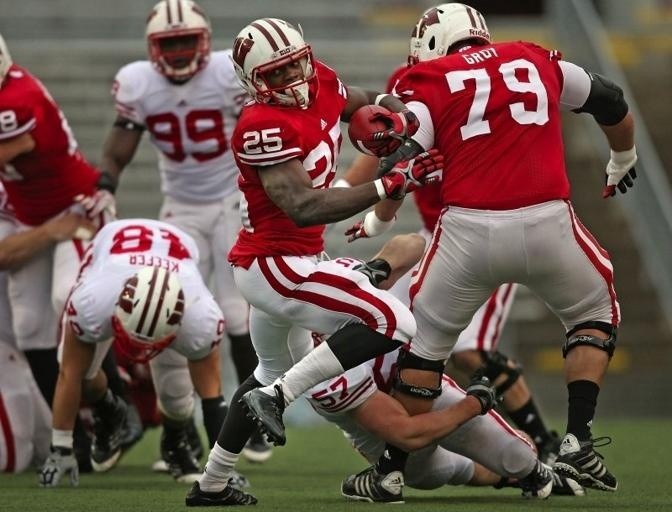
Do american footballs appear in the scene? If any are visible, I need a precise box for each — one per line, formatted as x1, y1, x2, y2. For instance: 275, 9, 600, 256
347, 105, 393, 158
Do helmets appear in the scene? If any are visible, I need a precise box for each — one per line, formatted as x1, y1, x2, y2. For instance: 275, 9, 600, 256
410, 2, 493, 60
0, 36, 12, 83
113, 266, 186, 367
145, 1, 211, 83
231, 18, 319, 110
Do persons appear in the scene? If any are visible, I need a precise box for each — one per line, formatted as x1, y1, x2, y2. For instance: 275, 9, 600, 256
1, 193, 84, 473
339, 2, 638, 505
41, 219, 230, 486
74, 1, 272, 465
331, 63, 562, 465
1, 33, 143, 475
184, 18, 444, 507
287, 230, 584, 500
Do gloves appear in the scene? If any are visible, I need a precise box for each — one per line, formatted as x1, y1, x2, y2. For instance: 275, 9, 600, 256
467, 375, 504, 417
84, 191, 117, 218
365, 107, 419, 157
230, 469, 249, 490
38, 443, 81, 487
601, 159, 638, 198
346, 219, 370, 243
378, 147, 444, 196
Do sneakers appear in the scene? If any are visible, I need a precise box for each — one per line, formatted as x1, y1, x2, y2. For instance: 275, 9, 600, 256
245, 435, 272, 462
185, 480, 257, 507
341, 468, 405, 502
518, 428, 617, 499
71, 400, 142, 474
153, 424, 204, 482
236, 385, 286, 444
